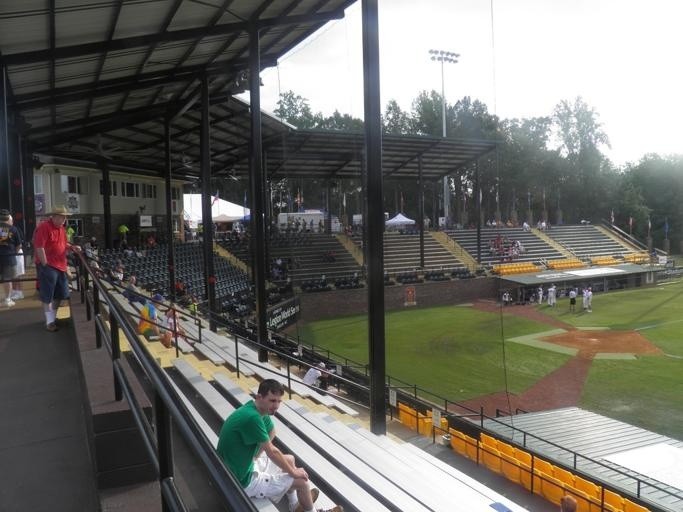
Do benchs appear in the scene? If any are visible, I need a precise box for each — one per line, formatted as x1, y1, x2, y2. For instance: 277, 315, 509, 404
88, 224, 682, 512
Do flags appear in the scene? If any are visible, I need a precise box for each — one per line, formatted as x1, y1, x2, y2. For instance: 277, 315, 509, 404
212, 192, 219, 206
610, 210, 669, 235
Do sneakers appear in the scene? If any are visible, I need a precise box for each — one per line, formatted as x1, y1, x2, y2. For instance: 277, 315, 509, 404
319, 505, 345, 512
45, 318, 60, 332
293, 488, 319, 512
5, 290, 24, 307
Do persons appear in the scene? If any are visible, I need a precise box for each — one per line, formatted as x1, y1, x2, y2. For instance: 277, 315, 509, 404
487, 231, 524, 262
397, 216, 592, 236
302, 361, 328, 396
77, 209, 363, 349
502, 283, 593, 314
558, 494, 577, 511
0, 208, 76, 309
215, 377, 344, 511
31, 203, 82, 332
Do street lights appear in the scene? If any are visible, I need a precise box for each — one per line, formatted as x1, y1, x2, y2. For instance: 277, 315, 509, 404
429, 49, 460, 229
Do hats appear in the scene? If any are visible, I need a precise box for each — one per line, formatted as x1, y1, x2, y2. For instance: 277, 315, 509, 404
45, 203, 73, 215
